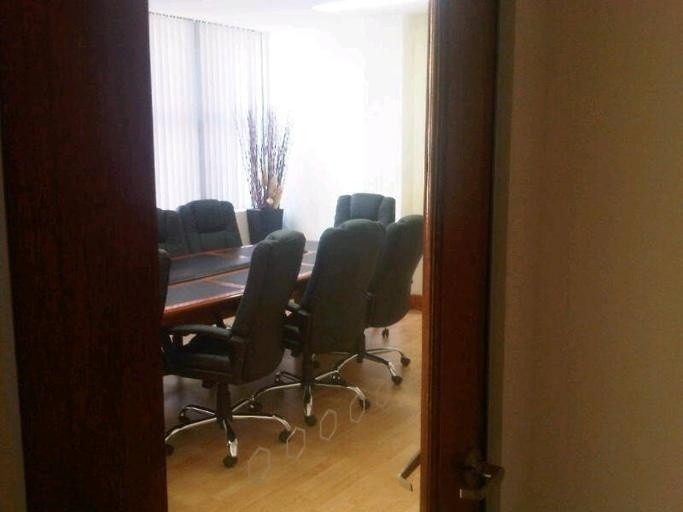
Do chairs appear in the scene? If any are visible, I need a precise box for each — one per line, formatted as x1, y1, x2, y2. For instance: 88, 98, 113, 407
324, 214, 425, 386
156, 205, 190, 255
330, 193, 399, 229
274, 218, 388, 428
162, 229, 308, 468
177, 197, 243, 251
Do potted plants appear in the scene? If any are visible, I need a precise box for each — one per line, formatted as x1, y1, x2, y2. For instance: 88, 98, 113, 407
234, 105, 294, 245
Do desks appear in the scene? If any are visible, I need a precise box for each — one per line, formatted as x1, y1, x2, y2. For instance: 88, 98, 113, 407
162, 238, 321, 335
